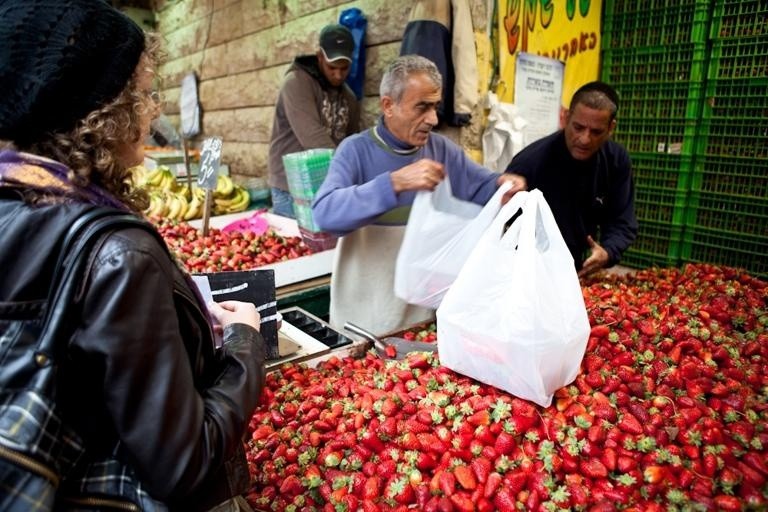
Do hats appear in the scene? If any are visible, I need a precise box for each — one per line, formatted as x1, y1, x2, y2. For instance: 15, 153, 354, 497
1, 1, 145, 140
319, 24, 355, 64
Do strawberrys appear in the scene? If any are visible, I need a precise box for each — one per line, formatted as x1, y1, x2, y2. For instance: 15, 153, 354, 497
237, 262, 767, 511
149, 216, 313, 274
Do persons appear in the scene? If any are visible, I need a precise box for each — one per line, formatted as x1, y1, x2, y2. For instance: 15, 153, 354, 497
503, 78, 641, 278
310, 50, 530, 341
266, 23, 363, 219
0, 0, 271, 512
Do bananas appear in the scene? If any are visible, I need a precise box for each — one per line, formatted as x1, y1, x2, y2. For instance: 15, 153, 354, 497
122, 166, 249, 221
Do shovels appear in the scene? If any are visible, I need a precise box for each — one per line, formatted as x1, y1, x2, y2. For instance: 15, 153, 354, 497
343, 322, 438, 362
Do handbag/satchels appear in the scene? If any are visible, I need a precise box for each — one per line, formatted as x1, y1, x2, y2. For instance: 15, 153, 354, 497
0, 300, 250, 511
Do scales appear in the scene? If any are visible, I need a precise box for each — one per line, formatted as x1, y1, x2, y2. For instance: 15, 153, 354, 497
146, 150, 199, 178
